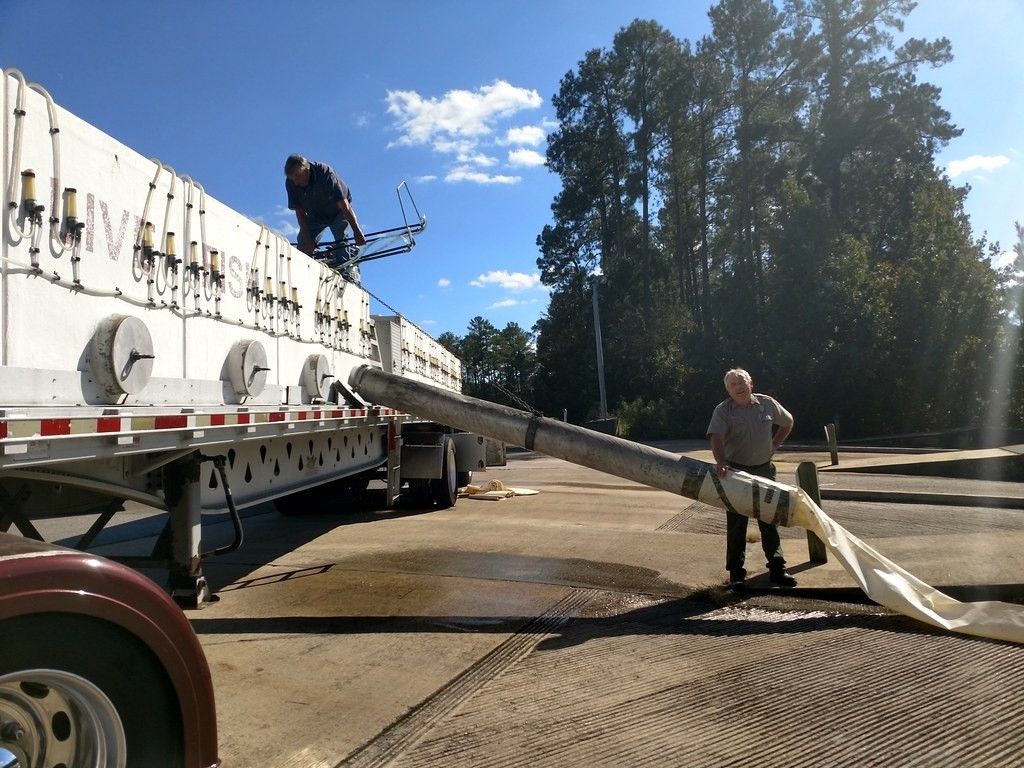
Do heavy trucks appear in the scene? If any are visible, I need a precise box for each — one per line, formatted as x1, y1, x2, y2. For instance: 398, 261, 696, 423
1, 66, 470, 613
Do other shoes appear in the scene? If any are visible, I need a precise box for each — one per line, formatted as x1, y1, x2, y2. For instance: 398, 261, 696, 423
731, 568, 747, 582
769, 572, 797, 586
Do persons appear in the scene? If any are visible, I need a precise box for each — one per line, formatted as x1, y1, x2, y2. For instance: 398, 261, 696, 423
284, 156, 367, 282
705, 368, 797, 589
